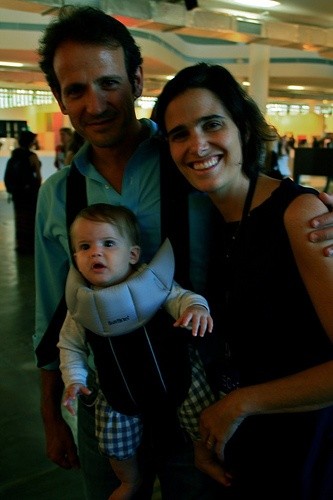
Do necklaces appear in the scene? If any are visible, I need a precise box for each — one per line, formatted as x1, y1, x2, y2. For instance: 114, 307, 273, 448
226, 176, 257, 279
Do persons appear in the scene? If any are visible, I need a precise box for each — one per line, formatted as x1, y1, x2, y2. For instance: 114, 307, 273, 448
32, 7, 333, 500
262, 129, 333, 179
56, 203, 232, 500
54, 127, 85, 170
151, 62, 333, 500
3, 131, 41, 257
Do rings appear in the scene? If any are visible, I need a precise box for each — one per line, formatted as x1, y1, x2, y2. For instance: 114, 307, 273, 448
207, 440, 214, 447
